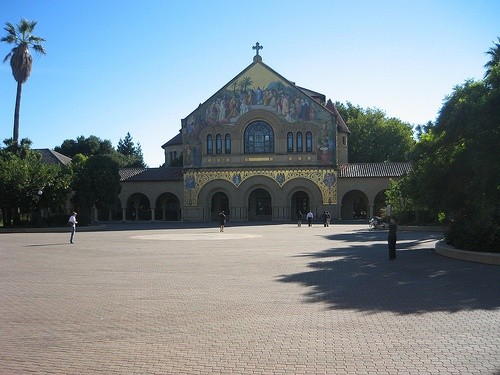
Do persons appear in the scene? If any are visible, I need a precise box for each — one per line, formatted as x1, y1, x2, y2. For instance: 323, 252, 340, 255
383, 217, 397, 260
297, 211, 303, 227
69, 212, 78, 244
307, 210, 313, 227
320, 211, 331, 227
219, 211, 226, 232
369, 218, 380, 229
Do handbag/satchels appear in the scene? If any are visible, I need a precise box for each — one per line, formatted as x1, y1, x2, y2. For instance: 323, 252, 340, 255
68, 222, 74, 226
224, 220, 227, 223
